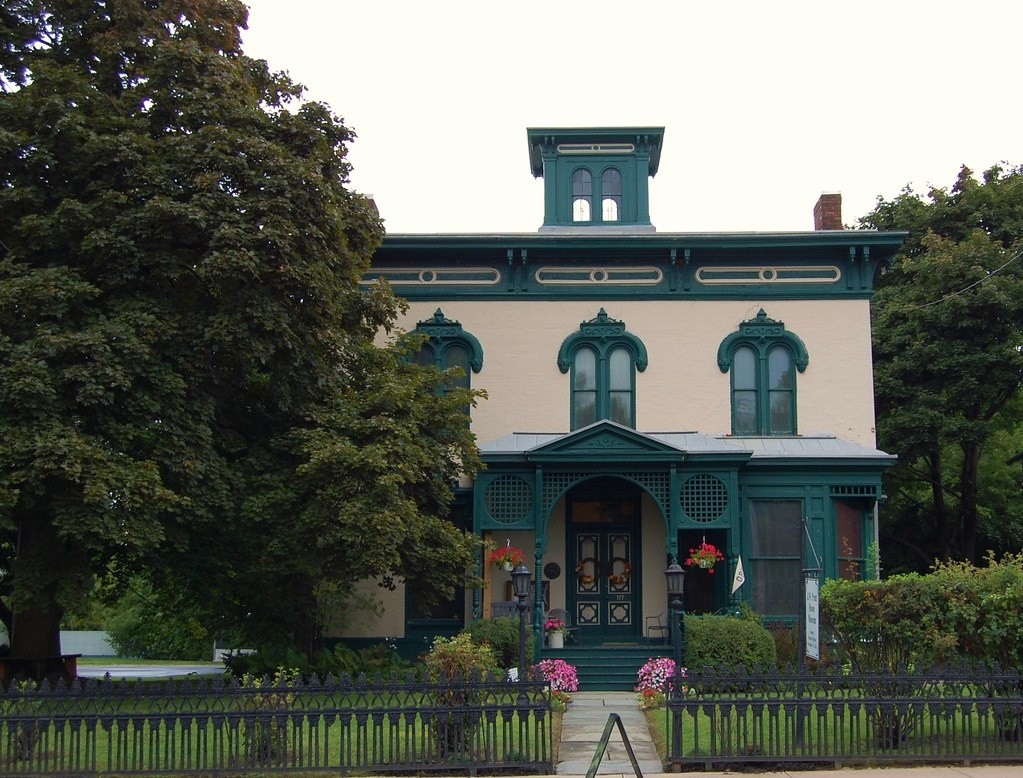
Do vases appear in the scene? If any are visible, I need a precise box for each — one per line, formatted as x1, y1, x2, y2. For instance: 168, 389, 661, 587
698, 558, 711, 568
547, 629, 564, 649
500, 560, 516, 573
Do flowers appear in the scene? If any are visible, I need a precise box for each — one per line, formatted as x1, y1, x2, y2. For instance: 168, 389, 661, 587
487, 539, 527, 571
527, 659, 580, 703
685, 535, 725, 574
610, 562, 631, 585
633, 657, 689, 710
575, 560, 595, 584
543, 617, 578, 650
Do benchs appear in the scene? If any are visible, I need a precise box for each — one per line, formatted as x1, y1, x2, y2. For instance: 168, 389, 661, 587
61, 652, 83, 677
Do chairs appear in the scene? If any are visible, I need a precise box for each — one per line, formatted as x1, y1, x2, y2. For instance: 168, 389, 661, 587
645, 608, 670, 645
